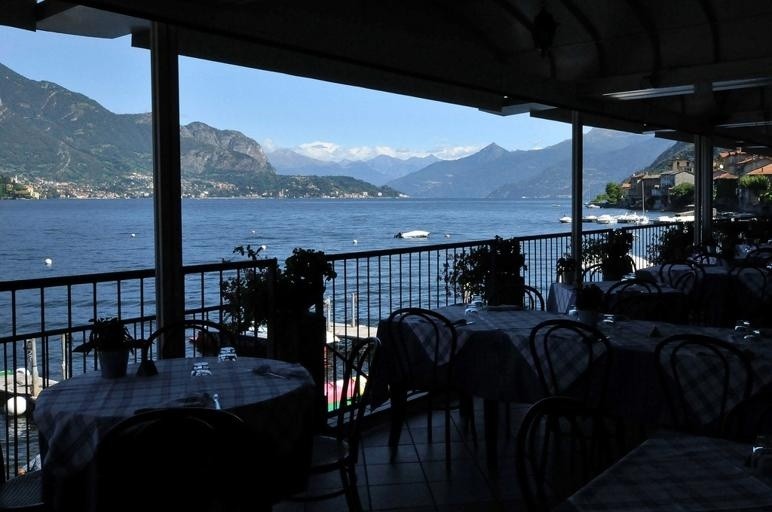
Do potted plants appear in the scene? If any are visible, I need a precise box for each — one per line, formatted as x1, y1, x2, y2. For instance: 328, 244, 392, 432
218, 246, 338, 399
73, 317, 151, 379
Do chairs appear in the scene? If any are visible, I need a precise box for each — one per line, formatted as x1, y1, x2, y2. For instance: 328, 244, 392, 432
96, 320, 379, 507
387, 215, 770, 511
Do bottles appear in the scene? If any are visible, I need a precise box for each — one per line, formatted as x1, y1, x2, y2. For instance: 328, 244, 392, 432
464, 296, 484, 325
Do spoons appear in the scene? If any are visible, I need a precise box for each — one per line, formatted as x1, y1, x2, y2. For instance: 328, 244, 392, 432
212, 393, 223, 410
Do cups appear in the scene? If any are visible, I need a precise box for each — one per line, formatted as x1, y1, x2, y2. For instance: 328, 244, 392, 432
191, 346, 238, 376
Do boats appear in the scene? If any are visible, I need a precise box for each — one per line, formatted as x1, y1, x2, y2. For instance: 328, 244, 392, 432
559, 216, 572, 223
396, 229, 431, 239
189, 317, 340, 381
582, 212, 695, 226
586, 204, 602, 210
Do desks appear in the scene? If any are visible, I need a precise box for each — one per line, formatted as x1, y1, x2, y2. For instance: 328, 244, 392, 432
39, 356, 317, 511
375, 308, 770, 465
